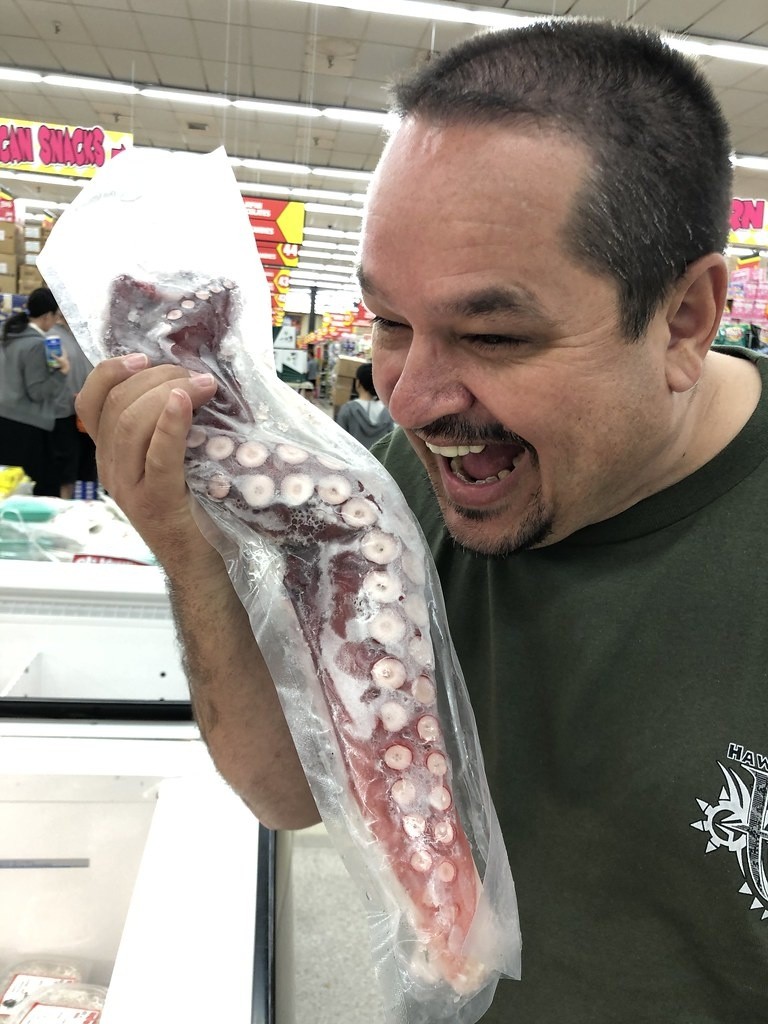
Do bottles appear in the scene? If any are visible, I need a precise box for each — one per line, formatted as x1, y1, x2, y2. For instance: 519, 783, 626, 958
72, 479, 104, 500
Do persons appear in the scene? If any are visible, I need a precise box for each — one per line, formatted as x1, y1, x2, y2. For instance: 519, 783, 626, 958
336, 363, 394, 450
75, 20, 768, 1024
0, 288, 98, 500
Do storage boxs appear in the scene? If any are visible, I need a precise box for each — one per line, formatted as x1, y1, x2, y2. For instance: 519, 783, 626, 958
331, 353, 370, 408
274, 320, 309, 382
2, 217, 61, 321
